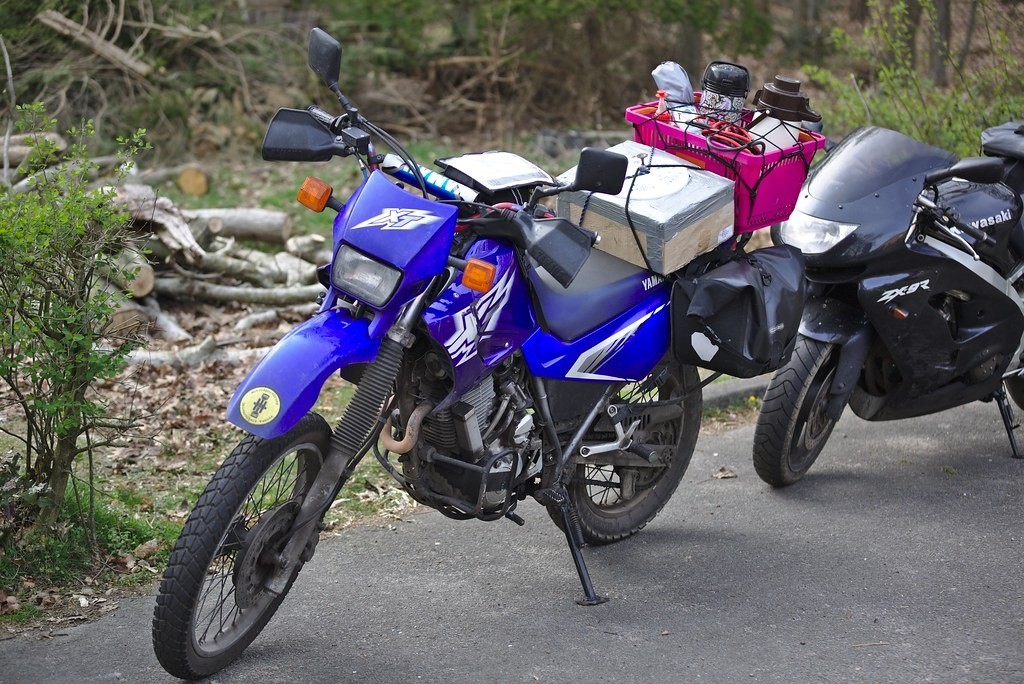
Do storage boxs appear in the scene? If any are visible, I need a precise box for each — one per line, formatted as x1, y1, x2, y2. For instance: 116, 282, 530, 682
625, 91, 826, 233
555, 139, 736, 276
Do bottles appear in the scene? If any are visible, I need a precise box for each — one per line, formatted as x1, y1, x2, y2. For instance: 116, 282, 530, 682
748, 75, 821, 155
700, 61, 749, 132
655, 91, 671, 126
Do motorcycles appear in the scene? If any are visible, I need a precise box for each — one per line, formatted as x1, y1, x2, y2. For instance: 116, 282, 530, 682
152, 26, 753, 684
755, 121, 1024, 491
